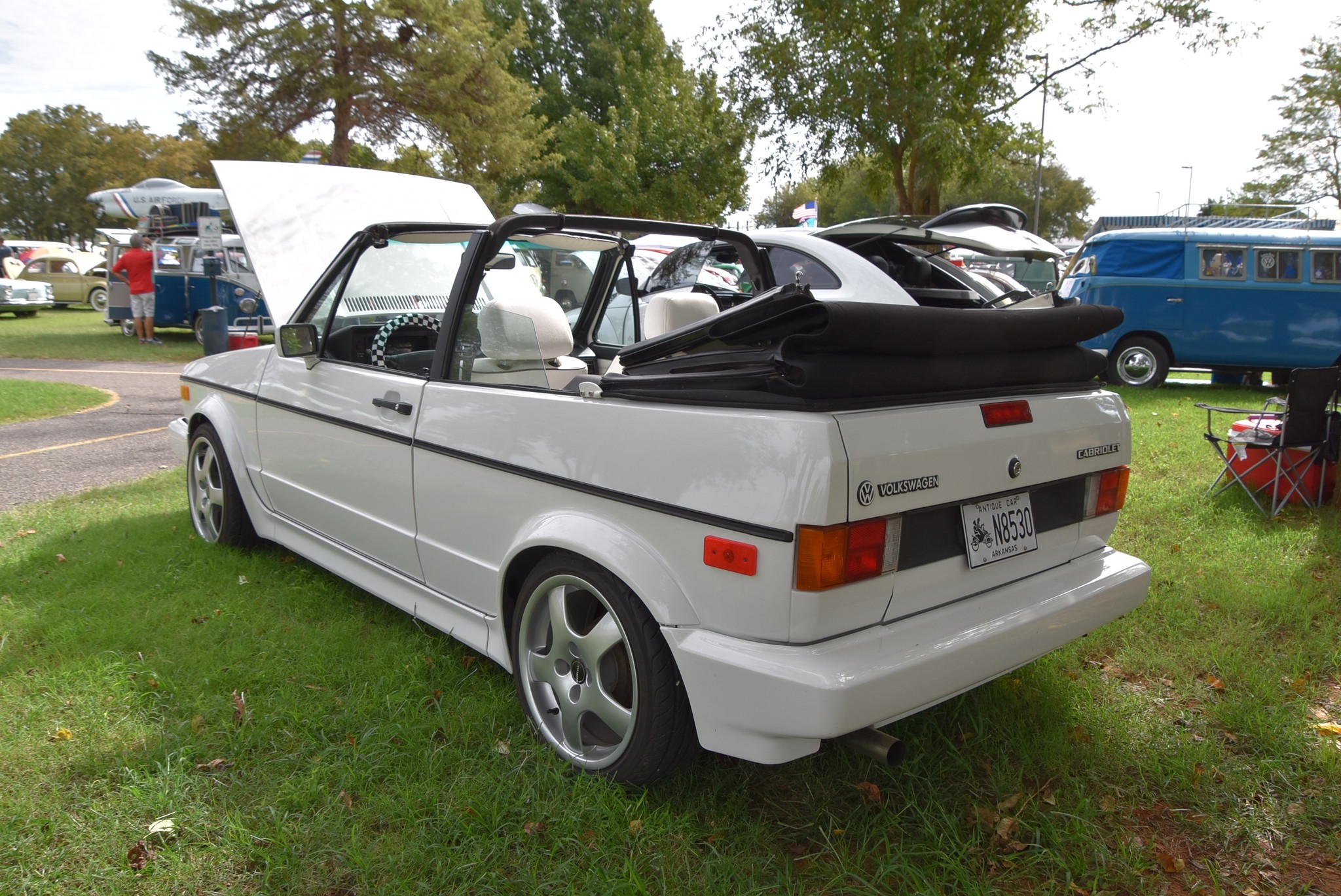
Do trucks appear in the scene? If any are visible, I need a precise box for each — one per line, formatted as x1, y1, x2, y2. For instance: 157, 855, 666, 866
528, 251, 622, 313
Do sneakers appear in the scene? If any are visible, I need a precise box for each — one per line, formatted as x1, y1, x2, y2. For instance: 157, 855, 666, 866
138, 337, 165, 345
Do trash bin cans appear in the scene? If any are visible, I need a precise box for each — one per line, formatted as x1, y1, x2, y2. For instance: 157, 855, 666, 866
199, 306, 230, 356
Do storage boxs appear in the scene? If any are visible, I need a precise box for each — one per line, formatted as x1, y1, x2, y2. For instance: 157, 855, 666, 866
143, 201, 221, 237
1226, 415, 1337, 509
228, 331, 259, 351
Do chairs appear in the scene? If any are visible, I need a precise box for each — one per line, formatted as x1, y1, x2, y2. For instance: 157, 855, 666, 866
458, 295, 589, 392
1194, 364, 1341, 522
604, 291, 720, 377
863, 254, 890, 276
901, 255, 944, 308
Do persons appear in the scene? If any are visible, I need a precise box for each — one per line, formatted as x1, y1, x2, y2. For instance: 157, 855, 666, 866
0, 237, 15, 278
111, 233, 166, 344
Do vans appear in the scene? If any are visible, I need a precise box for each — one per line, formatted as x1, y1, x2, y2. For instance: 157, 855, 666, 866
103, 233, 277, 347
1058, 204, 1341, 391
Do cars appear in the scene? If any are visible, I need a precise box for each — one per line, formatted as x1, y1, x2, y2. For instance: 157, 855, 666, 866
2, 250, 111, 313
167, 212, 1151, 785
562, 201, 1082, 346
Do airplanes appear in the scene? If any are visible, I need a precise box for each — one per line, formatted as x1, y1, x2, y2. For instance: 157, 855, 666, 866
83, 149, 323, 229
